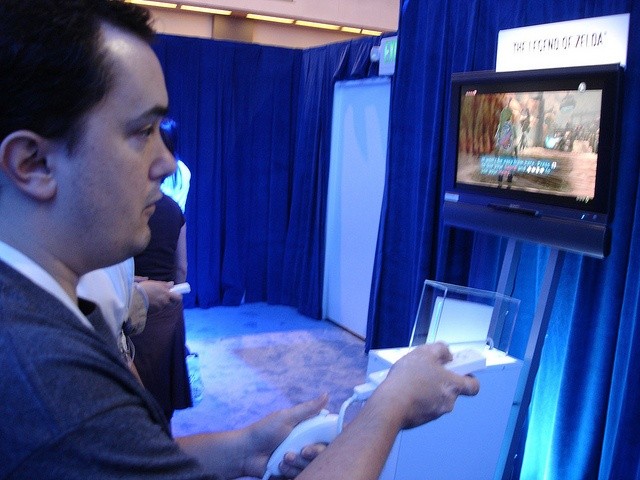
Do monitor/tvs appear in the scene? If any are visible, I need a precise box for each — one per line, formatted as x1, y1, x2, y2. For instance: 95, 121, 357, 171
436, 63, 626, 260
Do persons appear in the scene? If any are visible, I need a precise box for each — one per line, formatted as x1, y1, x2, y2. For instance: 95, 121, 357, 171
75, 257, 182, 389
125, 189, 192, 424
0, 1, 480, 479
155, 119, 192, 216
